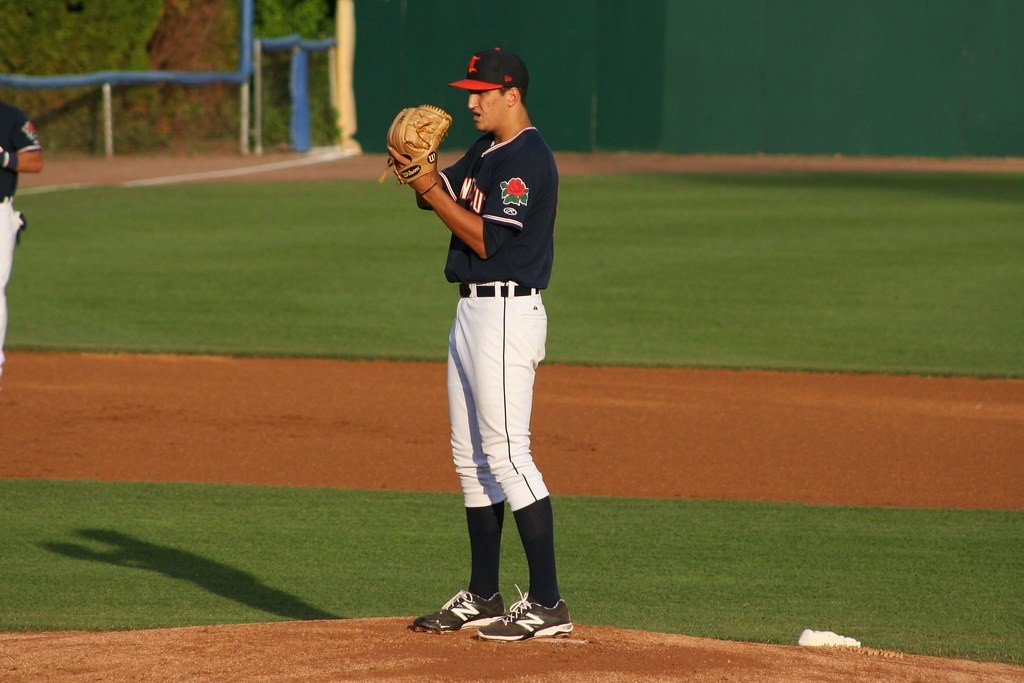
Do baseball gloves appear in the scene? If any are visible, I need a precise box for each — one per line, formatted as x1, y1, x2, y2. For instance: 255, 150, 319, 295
385, 103, 453, 186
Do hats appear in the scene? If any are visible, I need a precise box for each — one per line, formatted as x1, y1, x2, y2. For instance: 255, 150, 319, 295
446, 47, 528, 91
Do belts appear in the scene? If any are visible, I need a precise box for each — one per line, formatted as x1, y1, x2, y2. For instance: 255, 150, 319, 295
459, 283, 539, 298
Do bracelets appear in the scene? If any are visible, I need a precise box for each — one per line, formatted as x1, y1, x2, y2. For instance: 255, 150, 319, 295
421, 183, 437, 196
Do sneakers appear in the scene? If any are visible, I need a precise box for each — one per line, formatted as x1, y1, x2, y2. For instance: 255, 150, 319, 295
414, 591, 505, 634
477, 583, 573, 640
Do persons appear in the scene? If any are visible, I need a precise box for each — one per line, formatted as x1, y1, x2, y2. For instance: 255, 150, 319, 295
379, 47, 575, 642
0, 100, 44, 382
